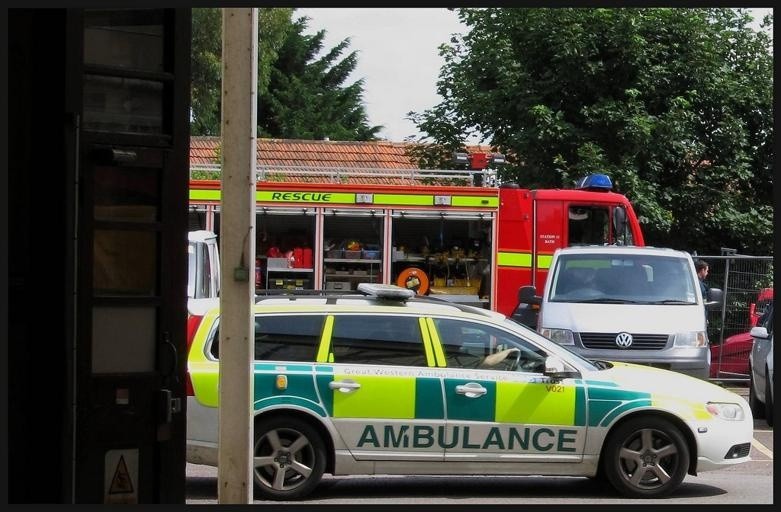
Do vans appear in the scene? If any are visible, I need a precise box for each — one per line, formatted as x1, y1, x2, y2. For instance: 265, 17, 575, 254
517, 245, 724, 382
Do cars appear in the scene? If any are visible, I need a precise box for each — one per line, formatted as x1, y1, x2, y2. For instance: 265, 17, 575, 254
181, 281, 754, 496
747, 297, 774, 428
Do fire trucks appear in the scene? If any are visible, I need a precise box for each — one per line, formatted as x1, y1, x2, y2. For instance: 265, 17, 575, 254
187, 134, 645, 331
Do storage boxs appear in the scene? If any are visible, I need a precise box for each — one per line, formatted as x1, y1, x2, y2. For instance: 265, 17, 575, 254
327, 249, 380, 260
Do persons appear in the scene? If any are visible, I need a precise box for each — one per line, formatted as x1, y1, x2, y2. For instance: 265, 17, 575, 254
694, 260, 711, 328
439, 323, 521, 370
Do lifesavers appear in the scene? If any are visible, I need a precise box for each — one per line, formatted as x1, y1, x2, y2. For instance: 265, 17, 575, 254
397, 268, 428, 296
